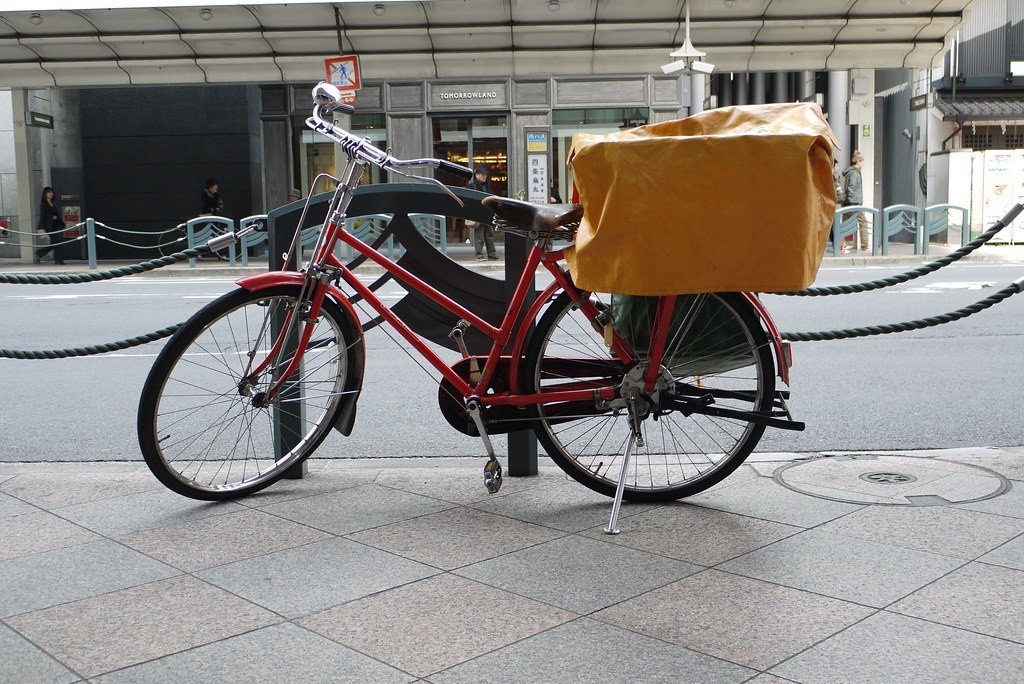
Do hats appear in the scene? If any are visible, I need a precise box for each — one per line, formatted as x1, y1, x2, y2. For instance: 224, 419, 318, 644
289, 189, 302, 198
850, 150, 864, 162
476, 166, 488, 173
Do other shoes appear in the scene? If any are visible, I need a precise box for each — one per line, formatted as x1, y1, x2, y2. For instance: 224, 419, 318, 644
36, 256, 40, 264
197, 255, 203, 261
840, 249, 850, 254
862, 249, 872, 253
54, 260, 66, 264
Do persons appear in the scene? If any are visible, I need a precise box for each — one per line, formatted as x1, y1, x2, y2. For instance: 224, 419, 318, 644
194, 178, 230, 261
288, 189, 302, 203
829, 157, 854, 250
836, 150, 873, 254
536, 178, 563, 251
464, 166, 503, 262
34, 186, 69, 265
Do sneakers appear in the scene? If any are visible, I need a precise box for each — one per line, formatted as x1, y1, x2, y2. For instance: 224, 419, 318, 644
477, 255, 502, 261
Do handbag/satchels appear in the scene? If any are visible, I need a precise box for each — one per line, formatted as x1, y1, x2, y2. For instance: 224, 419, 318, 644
465, 218, 481, 228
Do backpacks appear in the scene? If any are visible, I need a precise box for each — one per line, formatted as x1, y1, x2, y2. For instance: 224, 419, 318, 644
835, 168, 859, 203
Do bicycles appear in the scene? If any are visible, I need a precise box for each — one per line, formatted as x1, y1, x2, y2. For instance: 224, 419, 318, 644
139, 81, 806, 536
